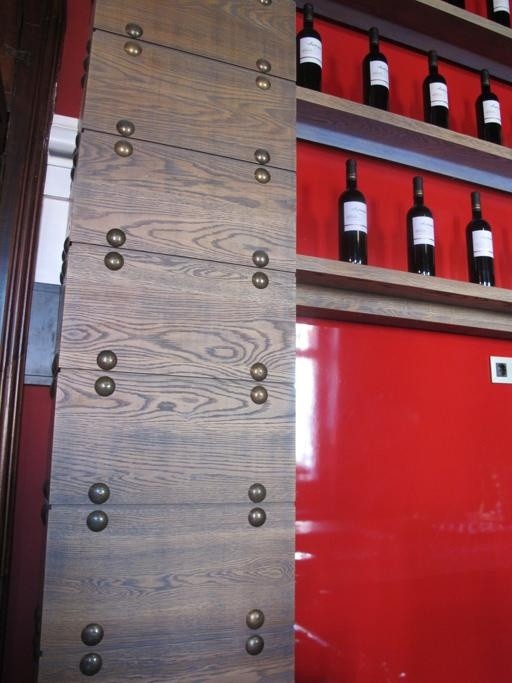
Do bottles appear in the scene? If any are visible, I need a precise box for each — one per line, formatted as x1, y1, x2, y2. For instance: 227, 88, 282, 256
422, 50, 449, 128
298, 2, 322, 91
464, 191, 496, 285
487, 1, 509, 27
475, 69, 502, 145
361, 26, 389, 110
404, 176, 437, 277
338, 159, 369, 265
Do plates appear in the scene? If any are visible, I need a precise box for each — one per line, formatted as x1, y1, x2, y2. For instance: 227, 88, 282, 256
295, 0, 512, 334
